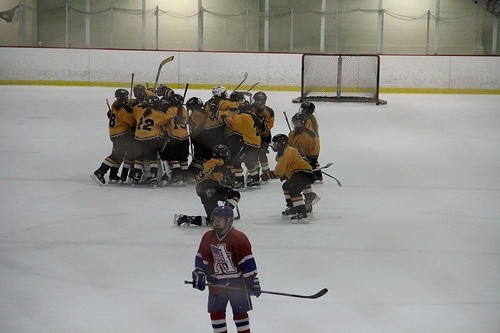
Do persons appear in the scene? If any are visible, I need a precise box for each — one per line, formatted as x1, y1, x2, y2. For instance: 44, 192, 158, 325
192, 206, 261, 333
289, 112, 320, 212
90, 84, 274, 191
301, 102, 322, 184
261, 134, 313, 223
174, 145, 241, 226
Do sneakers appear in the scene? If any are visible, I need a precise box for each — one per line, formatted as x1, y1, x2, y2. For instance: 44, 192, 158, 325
291, 204, 309, 224
91, 169, 106, 186
110, 169, 262, 192
304, 192, 320, 205
173, 213, 191, 226
315, 175, 323, 183
305, 205, 313, 213
282, 201, 296, 215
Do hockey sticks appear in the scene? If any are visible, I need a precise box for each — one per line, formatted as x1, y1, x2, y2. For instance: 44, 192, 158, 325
182, 82, 189, 105
231, 72, 247, 95
129, 72, 134, 99
283, 111, 292, 132
264, 141, 342, 188
310, 162, 334, 174
154, 56, 175, 89
247, 82, 259, 93
184, 279, 328, 299
233, 204, 240, 220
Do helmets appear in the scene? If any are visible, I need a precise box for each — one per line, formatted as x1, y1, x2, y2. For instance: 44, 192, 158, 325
115, 88, 129, 100
133, 83, 252, 114
273, 134, 289, 152
211, 206, 235, 233
301, 102, 316, 115
292, 113, 308, 133
253, 92, 267, 107
212, 144, 232, 162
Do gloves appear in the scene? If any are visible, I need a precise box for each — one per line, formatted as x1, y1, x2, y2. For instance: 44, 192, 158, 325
261, 170, 275, 181
246, 275, 262, 297
193, 267, 207, 290
